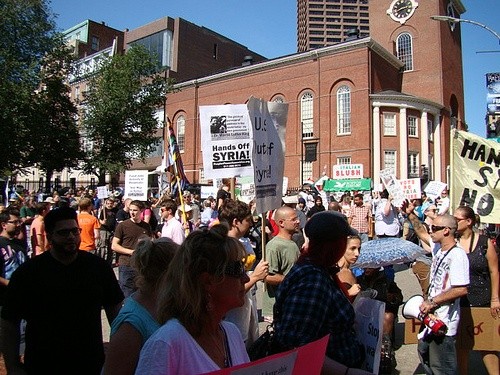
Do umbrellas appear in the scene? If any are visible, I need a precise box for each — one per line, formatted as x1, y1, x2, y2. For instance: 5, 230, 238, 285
349, 237, 429, 269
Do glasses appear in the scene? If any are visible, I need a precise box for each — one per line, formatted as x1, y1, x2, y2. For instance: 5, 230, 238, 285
432, 224, 452, 233
456, 218, 469, 223
5, 220, 21, 225
52, 228, 83, 238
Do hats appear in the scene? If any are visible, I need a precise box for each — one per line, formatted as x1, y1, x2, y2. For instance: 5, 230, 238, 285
178, 205, 193, 213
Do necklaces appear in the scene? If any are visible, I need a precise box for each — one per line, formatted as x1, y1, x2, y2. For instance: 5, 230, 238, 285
222, 330, 228, 369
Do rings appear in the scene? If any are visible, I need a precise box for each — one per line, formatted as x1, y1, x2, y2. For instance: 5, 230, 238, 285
497, 309, 499, 312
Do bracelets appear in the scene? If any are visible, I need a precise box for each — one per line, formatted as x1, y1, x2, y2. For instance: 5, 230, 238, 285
491, 297, 499, 302
345, 367, 349, 375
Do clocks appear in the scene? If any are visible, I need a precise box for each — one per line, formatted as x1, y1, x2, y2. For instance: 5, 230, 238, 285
392, 0, 412, 19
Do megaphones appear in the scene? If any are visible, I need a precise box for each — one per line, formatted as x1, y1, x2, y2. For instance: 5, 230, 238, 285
402, 294, 448, 336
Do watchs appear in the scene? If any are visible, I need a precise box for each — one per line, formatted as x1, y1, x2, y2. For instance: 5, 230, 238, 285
429, 298, 439, 309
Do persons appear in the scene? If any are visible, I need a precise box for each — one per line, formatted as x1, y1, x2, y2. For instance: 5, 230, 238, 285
0, 205, 125, 375
101, 237, 183, 375
0, 178, 480, 344
417, 215, 471, 375
135, 231, 251, 375
272, 210, 400, 375
453, 205, 500, 375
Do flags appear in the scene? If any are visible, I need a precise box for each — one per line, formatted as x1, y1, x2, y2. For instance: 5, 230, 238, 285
165, 118, 190, 195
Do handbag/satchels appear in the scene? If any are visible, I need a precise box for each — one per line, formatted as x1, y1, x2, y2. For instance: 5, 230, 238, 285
246, 323, 281, 360
